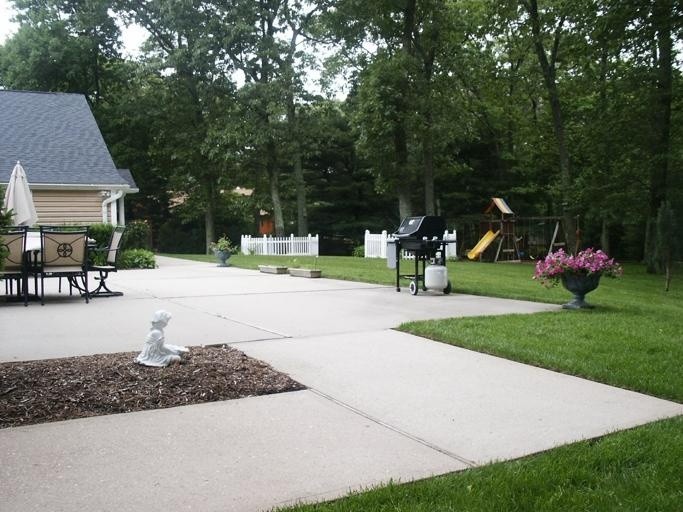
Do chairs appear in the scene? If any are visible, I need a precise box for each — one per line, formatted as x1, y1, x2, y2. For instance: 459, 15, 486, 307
0, 220, 127, 308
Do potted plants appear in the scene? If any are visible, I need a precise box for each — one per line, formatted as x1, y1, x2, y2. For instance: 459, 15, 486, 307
209, 234, 238, 267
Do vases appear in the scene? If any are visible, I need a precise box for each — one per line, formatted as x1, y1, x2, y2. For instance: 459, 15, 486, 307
557, 269, 602, 312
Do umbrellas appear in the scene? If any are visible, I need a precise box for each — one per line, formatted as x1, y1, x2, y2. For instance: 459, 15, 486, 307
0, 160, 38, 230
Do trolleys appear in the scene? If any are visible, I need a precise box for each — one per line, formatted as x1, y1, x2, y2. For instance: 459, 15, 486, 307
389, 214, 454, 294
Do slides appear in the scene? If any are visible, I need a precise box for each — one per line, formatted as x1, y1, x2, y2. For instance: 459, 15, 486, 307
467, 230, 499, 260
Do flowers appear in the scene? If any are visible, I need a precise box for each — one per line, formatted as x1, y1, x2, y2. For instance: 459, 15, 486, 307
526, 246, 625, 287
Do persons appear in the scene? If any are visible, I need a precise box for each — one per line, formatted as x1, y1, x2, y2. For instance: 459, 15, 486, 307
132, 310, 190, 365
